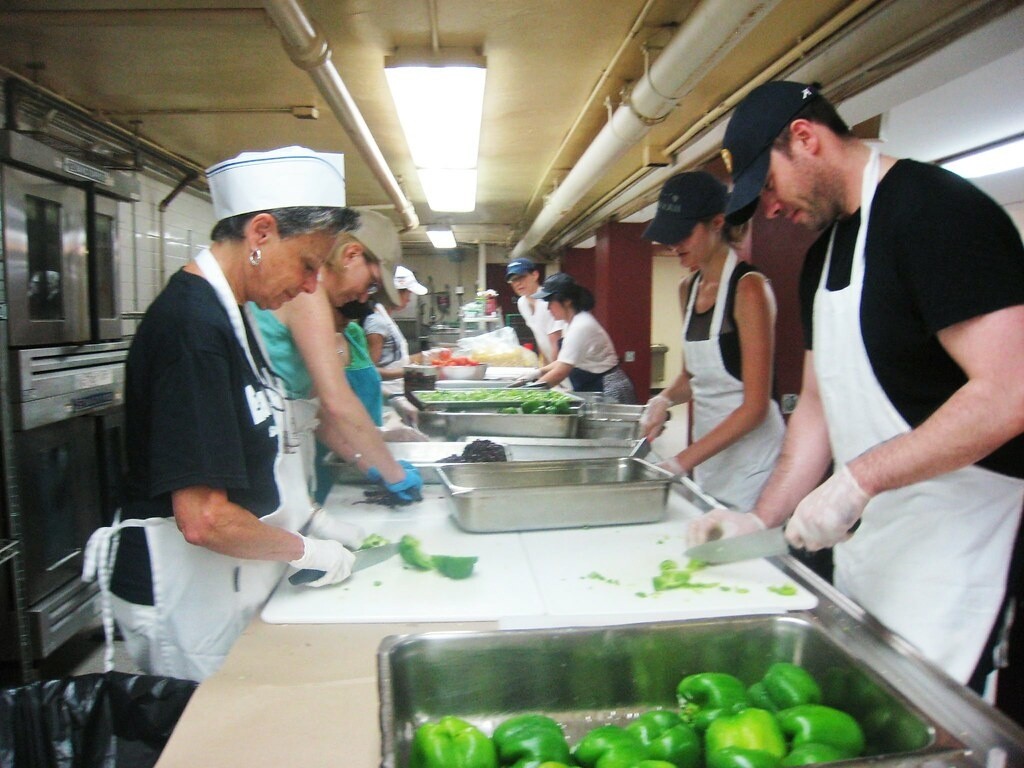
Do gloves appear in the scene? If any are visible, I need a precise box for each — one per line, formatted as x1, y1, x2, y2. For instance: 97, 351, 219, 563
366, 460, 423, 502
305, 505, 369, 549
289, 536, 353, 588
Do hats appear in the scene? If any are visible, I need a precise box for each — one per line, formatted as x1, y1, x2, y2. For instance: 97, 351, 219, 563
338, 210, 403, 307
720, 81, 819, 225
504, 258, 537, 279
393, 266, 428, 296
204, 147, 346, 221
639, 170, 721, 243
529, 272, 573, 299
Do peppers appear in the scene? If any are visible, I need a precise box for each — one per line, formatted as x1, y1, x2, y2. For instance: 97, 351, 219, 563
360, 535, 478, 580
585, 546, 799, 594
403, 659, 865, 768
497, 399, 573, 414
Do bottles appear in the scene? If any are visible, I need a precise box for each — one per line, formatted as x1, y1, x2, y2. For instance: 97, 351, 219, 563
485, 293, 495, 314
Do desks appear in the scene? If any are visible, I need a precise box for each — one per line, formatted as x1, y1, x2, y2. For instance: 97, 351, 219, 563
153, 348, 1024, 768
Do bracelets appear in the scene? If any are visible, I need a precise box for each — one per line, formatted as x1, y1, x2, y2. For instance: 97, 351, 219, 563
311, 502, 322, 514
350, 453, 363, 466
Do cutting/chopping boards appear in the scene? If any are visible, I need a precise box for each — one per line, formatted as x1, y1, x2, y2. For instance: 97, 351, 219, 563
260, 478, 819, 623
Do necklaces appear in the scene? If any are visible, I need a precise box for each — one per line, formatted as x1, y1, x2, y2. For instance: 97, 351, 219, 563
338, 349, 344, 356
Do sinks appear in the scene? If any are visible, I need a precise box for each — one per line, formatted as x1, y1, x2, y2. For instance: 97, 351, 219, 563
378, 611, 1024, 768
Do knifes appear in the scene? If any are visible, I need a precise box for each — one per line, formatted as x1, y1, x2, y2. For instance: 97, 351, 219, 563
687, 518, 861, 563
628, 412, 671, 458
289, 543, 401, 587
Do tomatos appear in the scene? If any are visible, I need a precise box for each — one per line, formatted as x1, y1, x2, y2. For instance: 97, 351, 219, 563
432, 350, 477, 366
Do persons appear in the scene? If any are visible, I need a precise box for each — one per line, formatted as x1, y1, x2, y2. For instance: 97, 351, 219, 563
333, 264, 448, 442
81, 145, 363, 683
638, 170, 788, 513
693, 79, 1024, 727
247, 205, 424, 501
507, 271, 637, 405
504, 258, 574, 390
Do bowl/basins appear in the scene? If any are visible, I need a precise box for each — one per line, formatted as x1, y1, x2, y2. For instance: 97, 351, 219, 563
438, 364, 488, 379
465, 312, 479, 318
402, 365, 440, 381
